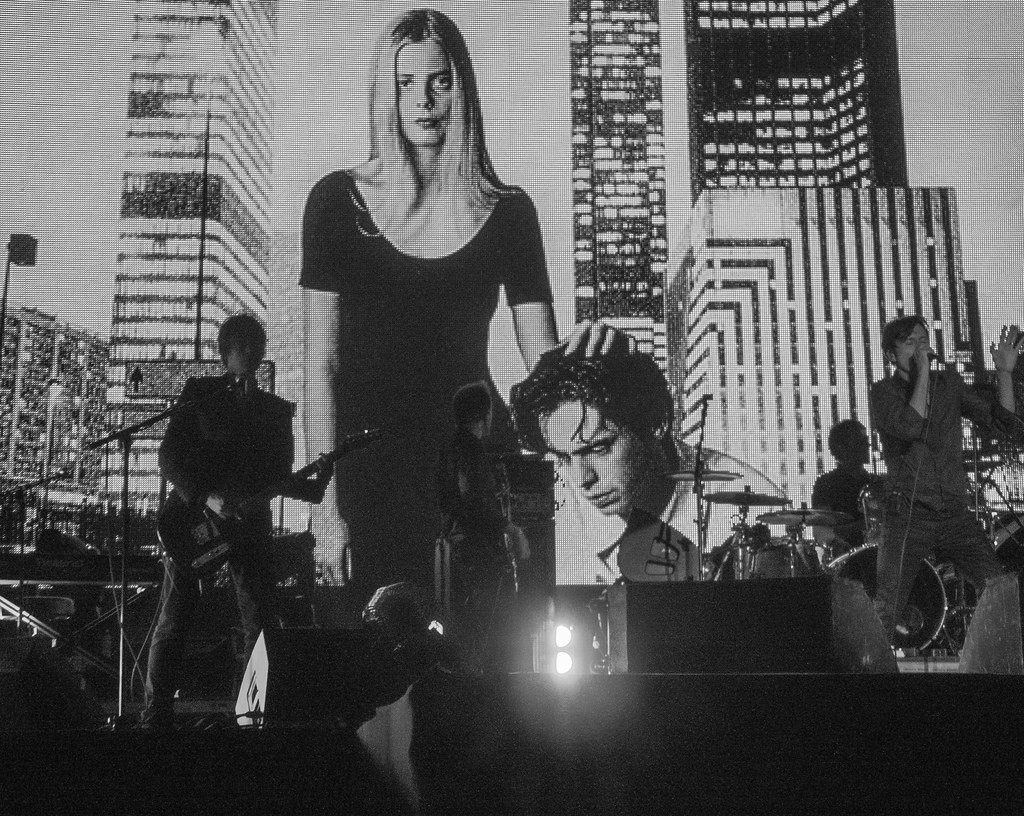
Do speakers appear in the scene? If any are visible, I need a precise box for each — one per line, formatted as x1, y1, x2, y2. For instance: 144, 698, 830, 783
229, 628, 411, 731
608, 573, 834, 674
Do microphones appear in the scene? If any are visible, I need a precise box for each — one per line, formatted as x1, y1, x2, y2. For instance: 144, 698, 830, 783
227, 373, 246, 386
926, 351, 942, 359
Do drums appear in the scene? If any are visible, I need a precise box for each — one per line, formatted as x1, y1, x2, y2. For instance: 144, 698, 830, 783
856, 471, 890, 542
826, 542, 948, 653
753, 538, 823, 578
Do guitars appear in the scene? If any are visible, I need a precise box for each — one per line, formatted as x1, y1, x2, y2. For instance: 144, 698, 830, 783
156, 427, 395, 585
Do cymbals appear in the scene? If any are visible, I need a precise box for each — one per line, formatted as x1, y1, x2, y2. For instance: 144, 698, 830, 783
667, 469, 743, 481
704, 490, 792, 506
962, 447, 1006, 474
755, 508, 858, 528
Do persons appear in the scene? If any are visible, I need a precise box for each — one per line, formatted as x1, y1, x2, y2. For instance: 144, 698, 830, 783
810, 419, 887, 596
133, 306, 338, 734
297, 7, 638, 595
506, 332, 803, 583
25, 529, 117, 704
867, 312, 1024, 649
433, 378, 532, 690
